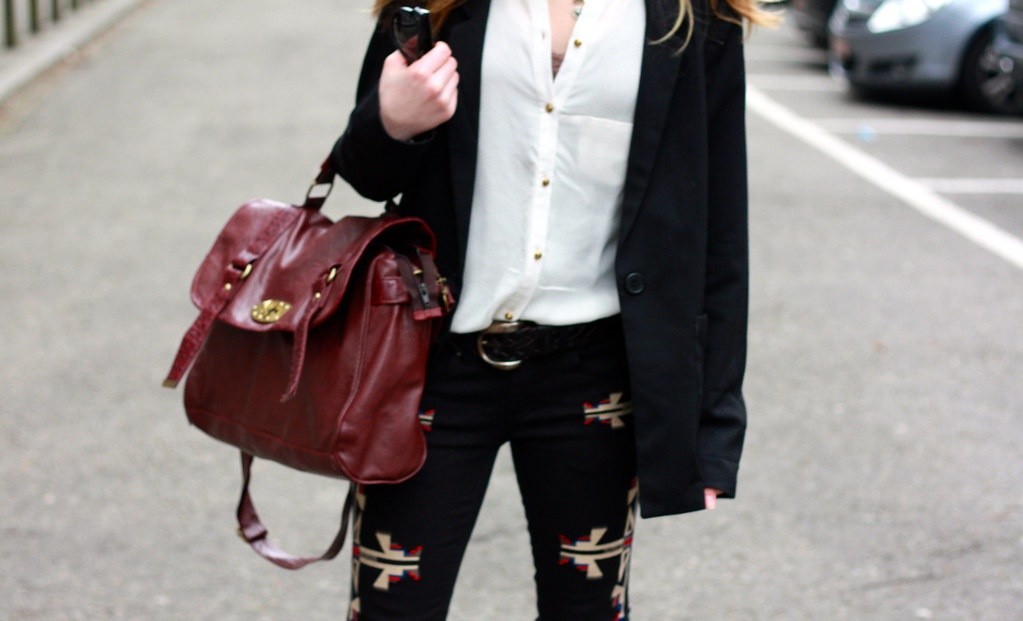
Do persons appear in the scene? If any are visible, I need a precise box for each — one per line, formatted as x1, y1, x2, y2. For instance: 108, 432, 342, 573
333, 0, 784, 621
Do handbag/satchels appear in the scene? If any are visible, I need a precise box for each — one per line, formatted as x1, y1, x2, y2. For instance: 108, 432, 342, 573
162, 157, 458, 571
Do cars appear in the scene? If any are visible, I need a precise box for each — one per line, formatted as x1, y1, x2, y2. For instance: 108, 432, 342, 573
828, 0, 1022, 116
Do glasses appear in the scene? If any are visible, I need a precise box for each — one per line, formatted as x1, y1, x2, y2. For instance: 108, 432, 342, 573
394, 7, 434, 144
